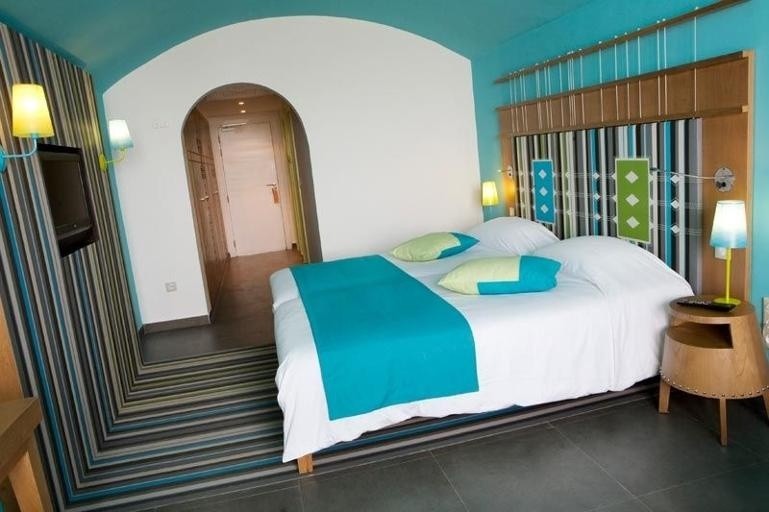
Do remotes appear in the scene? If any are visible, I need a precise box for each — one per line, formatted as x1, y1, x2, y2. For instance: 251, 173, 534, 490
677, 297, 736, 314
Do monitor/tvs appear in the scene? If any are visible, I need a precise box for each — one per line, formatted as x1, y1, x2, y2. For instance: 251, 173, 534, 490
35, 143, 100, 258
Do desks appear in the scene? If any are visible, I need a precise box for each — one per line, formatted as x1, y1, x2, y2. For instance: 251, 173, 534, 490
657, 296, 768, 448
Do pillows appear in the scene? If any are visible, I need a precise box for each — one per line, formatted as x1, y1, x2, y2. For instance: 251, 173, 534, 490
388, 232, 481, 262
437, 254, 562, 295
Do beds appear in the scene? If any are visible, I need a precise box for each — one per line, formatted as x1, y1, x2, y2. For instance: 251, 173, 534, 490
272, 235, 697, 475
269, 215, 561, 312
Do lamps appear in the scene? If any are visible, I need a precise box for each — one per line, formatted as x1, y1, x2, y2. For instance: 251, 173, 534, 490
649, 166, 734, 193
481, 181, 500, 218
0, 82, 56, 174
98, 119, 136, 172
498, 165, 514, 178
708, 200, 750, 305
